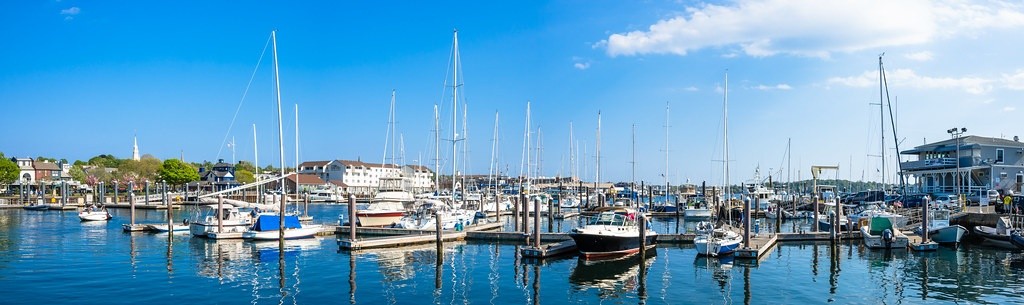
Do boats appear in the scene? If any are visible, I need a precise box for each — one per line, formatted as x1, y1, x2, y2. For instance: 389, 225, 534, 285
78, 211, 112, 222
860, 217, 909, 250
22, 202, 64, 212
146, 219, 189, 234
243, 212, 319, 240
564, 194, 658, 268
914, 200, 969, 250
973, 216, 1024, 253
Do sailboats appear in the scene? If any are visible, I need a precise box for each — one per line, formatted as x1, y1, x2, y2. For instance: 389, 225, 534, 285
692, 70, 744, 256
187, 26, 313, 238
357, 28, 920, 227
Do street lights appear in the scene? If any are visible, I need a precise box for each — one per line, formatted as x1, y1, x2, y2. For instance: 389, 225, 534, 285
947, 128, 968, 210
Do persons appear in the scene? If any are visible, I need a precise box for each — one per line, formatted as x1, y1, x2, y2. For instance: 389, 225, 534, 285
995, 183, 1004, 194
1004, 195, 1013, 213
